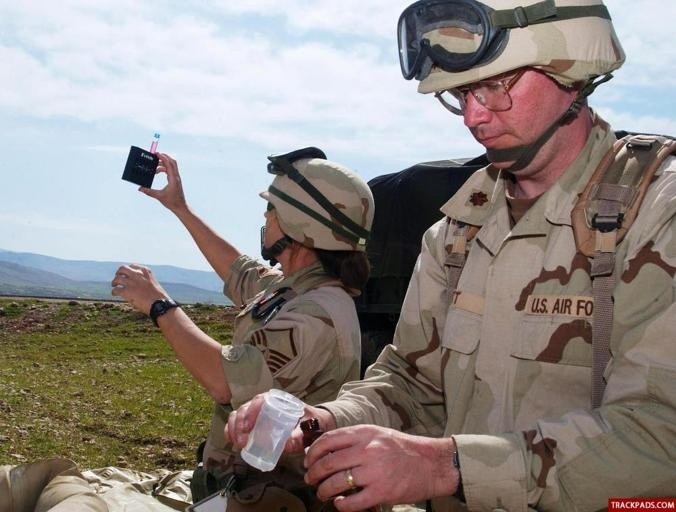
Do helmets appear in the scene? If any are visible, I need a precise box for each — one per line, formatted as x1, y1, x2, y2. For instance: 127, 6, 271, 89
418, 0, 626, 94
259, 158, 375, 252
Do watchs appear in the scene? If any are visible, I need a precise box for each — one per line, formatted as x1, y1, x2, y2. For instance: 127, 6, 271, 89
147, 297, 179, 329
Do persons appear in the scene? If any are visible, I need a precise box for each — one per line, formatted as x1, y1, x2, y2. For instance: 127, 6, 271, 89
220, 0, 676, 512
110, 146, 377, 512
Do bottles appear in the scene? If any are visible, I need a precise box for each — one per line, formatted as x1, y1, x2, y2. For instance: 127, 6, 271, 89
300, 418, 382, 512
150, 132, 160, 154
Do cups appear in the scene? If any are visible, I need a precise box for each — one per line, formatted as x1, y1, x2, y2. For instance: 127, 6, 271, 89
240, 387, 305, 472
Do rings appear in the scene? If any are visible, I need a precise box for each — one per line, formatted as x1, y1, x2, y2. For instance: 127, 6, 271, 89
344, 469, 357, 491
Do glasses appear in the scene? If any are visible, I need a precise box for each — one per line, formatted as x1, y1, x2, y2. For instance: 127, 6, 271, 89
398, 0, 510, 81
267, 202, 275, 210
434, 67, 529, 116
267, 147, 326, 175
